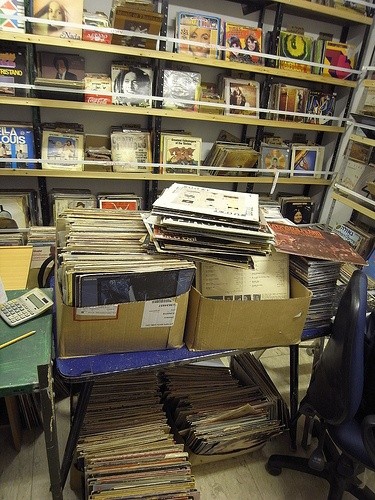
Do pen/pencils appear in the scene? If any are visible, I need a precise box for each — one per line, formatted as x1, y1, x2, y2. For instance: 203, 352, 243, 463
0, 330, 36, 348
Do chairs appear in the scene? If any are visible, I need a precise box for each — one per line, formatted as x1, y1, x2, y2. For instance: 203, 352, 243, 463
265, 269, 375, 500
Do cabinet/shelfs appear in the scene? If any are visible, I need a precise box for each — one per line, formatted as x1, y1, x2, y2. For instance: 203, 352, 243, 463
1, 0, 375, 290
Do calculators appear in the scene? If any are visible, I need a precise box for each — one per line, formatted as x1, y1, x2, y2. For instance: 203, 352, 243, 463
0, 287, 54, 327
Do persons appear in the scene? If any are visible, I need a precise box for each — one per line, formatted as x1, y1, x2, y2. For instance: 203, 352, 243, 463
112, 67, 151, 107
228, 36, 250, 62
0, 144, 7, 168
242, 35, 262, 64
60, 140, 75, 160
227, 295, 261, 301
17, 150, 26, 168
188, 25, 211, 57
53, 58, 77, 80
291, 210, 307, 224
32, 0, 75, 37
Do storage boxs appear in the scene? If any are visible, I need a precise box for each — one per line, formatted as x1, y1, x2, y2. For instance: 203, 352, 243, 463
184, 271, 314, 354
53, 229, 191, 360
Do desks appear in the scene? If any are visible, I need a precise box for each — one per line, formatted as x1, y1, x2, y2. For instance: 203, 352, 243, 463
0, 288, 63, 500
55, 320, 334, 491
